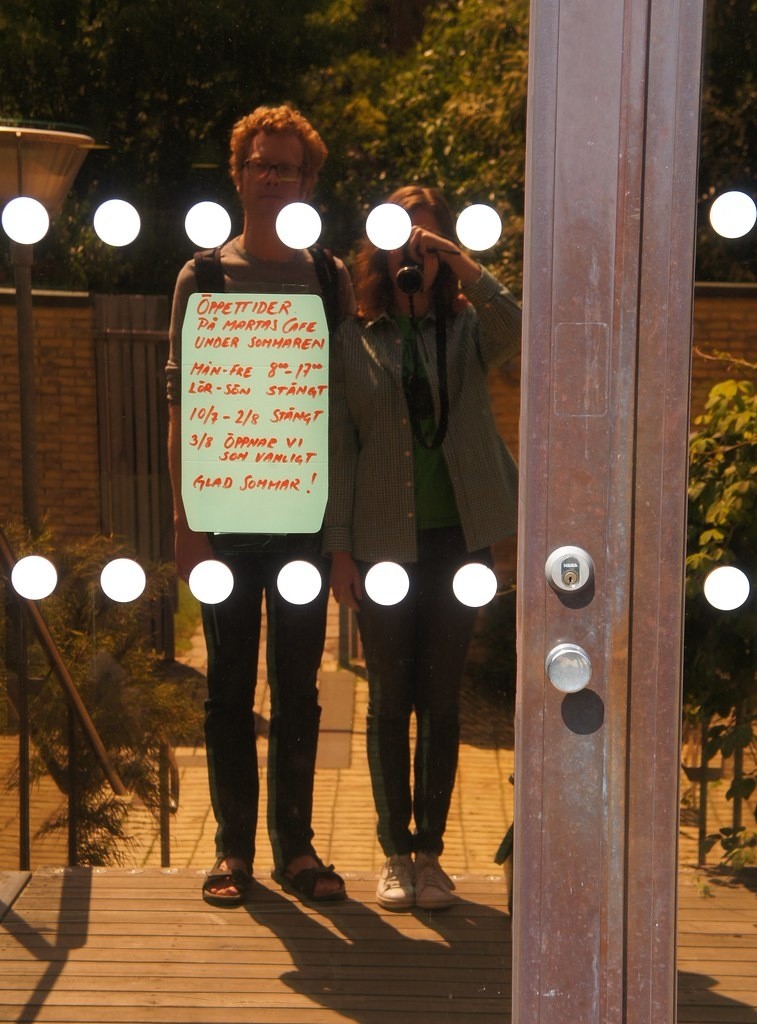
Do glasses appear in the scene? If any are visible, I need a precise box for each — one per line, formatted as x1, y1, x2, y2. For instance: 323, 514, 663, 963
242, 156, 307, 183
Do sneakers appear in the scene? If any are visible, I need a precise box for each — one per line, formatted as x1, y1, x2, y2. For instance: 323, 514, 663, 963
376, 853, 415, 910
410, 850, 456, 907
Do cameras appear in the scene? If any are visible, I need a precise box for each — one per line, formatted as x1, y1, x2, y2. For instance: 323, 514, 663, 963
396, 249, 426, 295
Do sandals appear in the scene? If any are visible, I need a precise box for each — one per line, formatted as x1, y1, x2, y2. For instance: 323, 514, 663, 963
202, 854, 254, 905
270, 844, 347, 903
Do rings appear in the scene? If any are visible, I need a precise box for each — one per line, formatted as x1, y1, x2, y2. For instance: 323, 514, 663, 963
185, 571, 190, 576
333, 593, 336, 597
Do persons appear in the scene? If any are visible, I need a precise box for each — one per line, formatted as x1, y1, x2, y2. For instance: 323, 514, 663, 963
163, 100, 354, 905
321, 182, 517, 909
